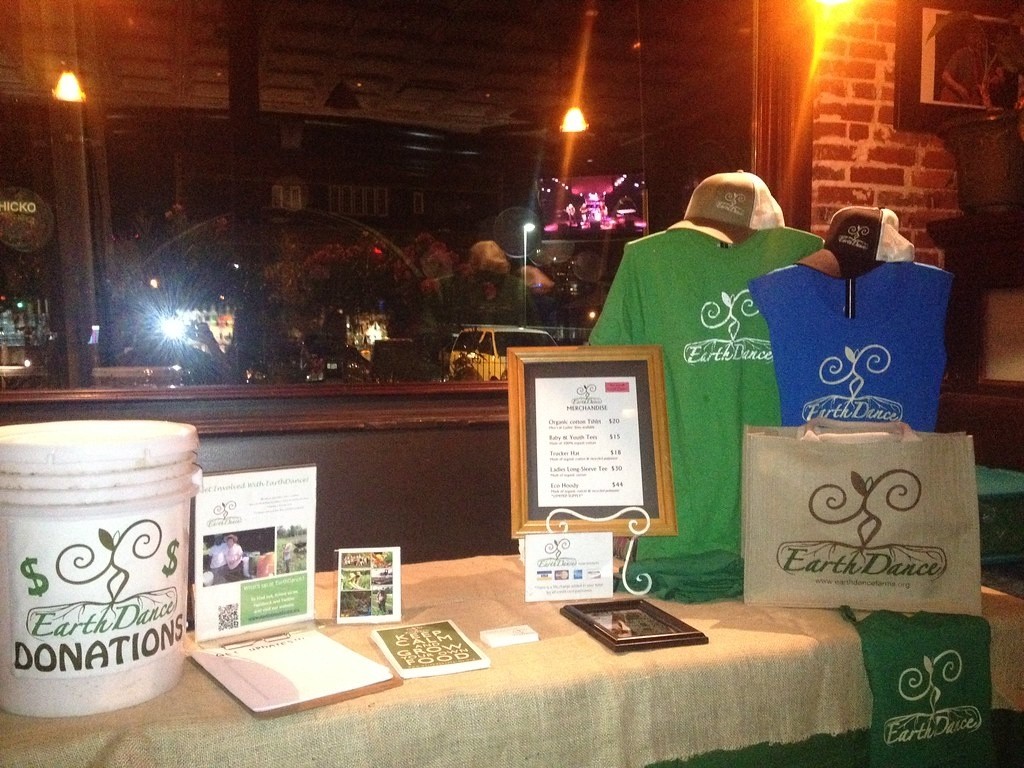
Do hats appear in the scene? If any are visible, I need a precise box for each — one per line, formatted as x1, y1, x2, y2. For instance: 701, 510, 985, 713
667, 170, 784, 244
793, 207, 916, 279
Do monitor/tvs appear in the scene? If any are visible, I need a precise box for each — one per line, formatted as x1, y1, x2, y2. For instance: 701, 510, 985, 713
538, 174, 647, 243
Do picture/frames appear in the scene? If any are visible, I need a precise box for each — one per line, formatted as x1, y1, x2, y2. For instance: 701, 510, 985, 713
560, 599, 711, 655
506, 345, 680, 540
894, 0, 1024, 132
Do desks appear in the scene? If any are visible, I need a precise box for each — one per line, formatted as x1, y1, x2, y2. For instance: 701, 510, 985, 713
0, 553, 1024, 768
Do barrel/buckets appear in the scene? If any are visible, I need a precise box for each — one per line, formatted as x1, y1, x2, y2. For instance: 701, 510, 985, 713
0, 421, 203, 717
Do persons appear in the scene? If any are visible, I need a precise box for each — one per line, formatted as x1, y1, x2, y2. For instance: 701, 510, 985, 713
377, 590, 387, 612
457, 239, 546, 357
208, 534, 243, 585
282, 544, 291, 573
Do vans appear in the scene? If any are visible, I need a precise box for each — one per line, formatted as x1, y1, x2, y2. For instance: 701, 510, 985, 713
449, 327, 560, 383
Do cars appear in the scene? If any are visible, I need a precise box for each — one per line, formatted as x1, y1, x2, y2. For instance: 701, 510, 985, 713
246, 340, 381, 388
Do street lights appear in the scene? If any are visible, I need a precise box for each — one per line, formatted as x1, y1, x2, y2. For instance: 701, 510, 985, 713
523, 223, 535, 327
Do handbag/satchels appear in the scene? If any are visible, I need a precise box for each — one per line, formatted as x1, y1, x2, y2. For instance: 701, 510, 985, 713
737, 416, 985, 620
